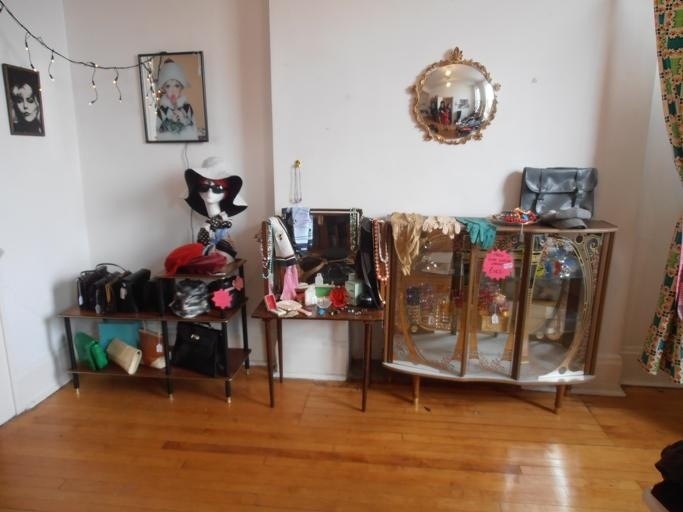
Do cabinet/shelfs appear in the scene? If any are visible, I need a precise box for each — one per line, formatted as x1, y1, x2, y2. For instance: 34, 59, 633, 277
56, 259, 251, 404
381, 216, 617, 415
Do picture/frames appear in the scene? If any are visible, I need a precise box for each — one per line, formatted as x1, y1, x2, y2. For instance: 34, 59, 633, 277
138, 52, 208, 143
2, 64, 45, 136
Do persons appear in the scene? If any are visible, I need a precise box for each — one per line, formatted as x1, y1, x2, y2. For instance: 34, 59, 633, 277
7, 67, 44, 136
150, 57, 200, 139
428, 99, 452, 126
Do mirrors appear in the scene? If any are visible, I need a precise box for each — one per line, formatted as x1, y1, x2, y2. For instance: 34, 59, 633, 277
411, 47, 502, 144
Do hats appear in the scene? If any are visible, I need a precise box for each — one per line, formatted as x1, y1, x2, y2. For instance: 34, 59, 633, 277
156, 59, 186, 89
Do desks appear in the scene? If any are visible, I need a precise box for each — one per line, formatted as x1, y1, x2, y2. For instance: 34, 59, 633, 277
251, 300, 384, 412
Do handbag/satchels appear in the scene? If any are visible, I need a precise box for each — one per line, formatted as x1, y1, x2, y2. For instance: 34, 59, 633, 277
519, 167, 598, 223
76, 263, 175, 314
73, 320, 225, 379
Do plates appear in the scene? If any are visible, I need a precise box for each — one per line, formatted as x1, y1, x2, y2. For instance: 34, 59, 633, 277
494, 214, 543, 225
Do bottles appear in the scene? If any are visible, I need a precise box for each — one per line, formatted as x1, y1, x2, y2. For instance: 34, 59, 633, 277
405, 282, 451, 332
